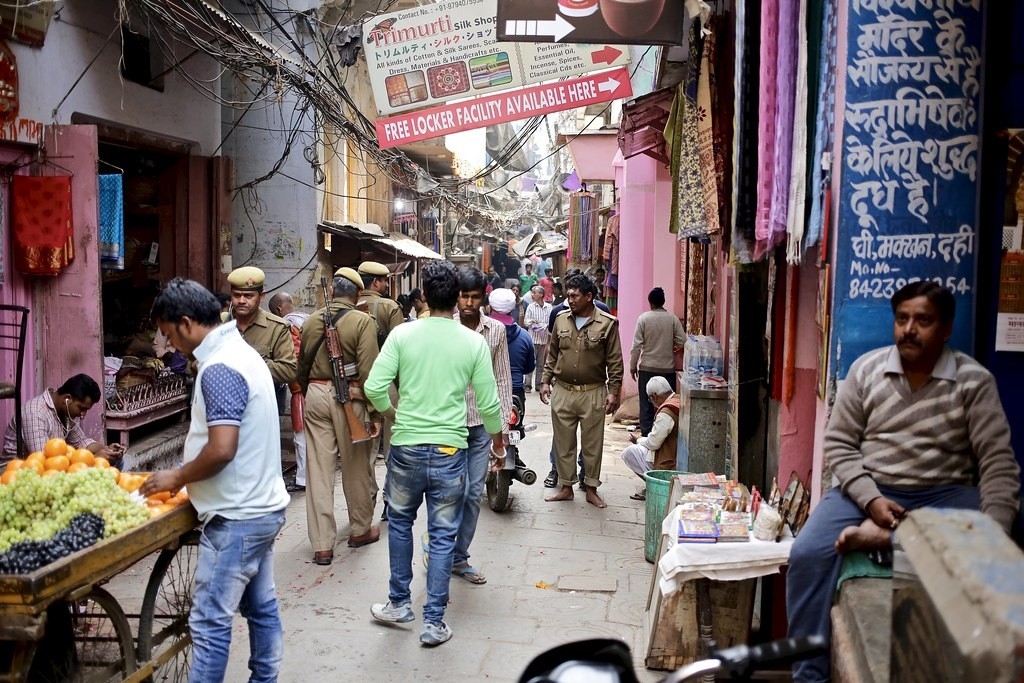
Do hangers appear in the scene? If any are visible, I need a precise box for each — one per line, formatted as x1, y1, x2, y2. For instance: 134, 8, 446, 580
8, 146, 74, 178
98, 158, 124, 176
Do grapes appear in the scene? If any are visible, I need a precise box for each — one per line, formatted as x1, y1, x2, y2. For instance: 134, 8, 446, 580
0, 465, 150, 576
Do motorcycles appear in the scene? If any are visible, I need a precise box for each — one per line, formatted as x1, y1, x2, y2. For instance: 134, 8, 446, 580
485, 395, 537, 514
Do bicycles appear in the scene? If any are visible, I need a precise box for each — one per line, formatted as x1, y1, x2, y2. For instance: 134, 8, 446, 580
516, 636, 828, 683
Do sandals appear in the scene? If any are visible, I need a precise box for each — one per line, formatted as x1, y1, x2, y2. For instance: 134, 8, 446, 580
545, 470, 558, 487
630, 488, 646, 500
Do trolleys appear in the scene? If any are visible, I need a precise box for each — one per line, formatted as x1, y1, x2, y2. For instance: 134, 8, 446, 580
0, 526, 203, 683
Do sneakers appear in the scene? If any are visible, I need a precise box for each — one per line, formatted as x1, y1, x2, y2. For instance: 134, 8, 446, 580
420, 621, 453, 644
370, 601, 415, 623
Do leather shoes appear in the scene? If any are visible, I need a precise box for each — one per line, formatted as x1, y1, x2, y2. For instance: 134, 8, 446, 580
348, 525, 380, 547
314, 550, 333, 565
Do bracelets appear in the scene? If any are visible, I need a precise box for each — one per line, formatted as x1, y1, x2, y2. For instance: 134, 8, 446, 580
493, 439, 506, 449
490, 443, 508, 458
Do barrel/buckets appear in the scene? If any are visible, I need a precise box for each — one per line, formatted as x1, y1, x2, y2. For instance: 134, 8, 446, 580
642, 469, 695, 563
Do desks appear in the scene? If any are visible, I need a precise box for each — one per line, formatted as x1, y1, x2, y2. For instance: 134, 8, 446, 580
106, 374, 189, 449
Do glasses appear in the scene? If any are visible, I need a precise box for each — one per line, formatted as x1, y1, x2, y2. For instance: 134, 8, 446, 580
648, 394, 653, 402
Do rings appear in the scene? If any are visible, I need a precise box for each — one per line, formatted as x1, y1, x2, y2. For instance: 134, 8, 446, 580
901, 509, 907, 514
890, 519, 896, 528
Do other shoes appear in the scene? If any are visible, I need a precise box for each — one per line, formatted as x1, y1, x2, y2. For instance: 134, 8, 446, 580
286, 484, 305, 492
524, 386, 533, 393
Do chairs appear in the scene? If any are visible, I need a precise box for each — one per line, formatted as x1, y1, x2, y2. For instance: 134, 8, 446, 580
0, 304, 30, 467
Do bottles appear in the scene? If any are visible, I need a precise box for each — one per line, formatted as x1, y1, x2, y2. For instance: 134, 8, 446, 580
682, 334, 725, 379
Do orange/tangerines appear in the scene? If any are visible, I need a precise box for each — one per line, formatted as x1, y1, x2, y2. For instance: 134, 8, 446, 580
0, 438, 188, 520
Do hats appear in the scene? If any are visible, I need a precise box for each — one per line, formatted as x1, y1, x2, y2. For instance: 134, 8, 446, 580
564, 269, 584, 279
488, 287, 517, 314
358, 261, 390, 276
334, 267, 365, 290
228, 266, 265, 290
648, 287, 665, 305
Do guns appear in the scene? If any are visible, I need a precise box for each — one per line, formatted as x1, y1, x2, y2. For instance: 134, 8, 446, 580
321, 276, 371, 445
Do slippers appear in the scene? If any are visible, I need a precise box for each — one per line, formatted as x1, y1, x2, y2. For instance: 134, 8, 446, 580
452, 564, 487, 584
422, 532, 429, 569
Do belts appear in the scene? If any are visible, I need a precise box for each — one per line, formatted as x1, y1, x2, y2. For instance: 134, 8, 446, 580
557, 378, 605, 392
308, 379, 362, 387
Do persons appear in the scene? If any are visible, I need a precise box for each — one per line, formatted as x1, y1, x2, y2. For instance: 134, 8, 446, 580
620, 376, 680, 500
363, 258, 508, 644
2, 373, 127, 473
785, 280, 1021, 683
268, 258, 624, 644
138, 277, 290, 683
185, 266, 299, 383
296, 266, 383, 565
538, 274, 625, 508
630, 287, 688, 437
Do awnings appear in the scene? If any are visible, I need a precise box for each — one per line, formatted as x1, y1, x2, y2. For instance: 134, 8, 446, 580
318, 224, 446, 261
